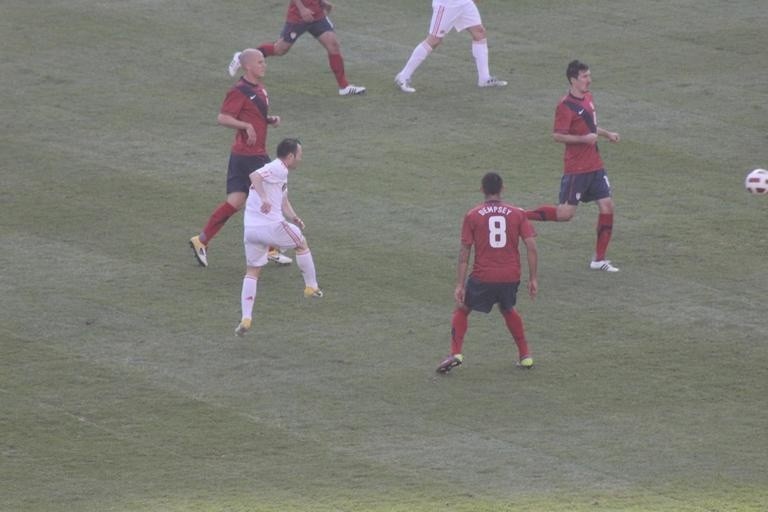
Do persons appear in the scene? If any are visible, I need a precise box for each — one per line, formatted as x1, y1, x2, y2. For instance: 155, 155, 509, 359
524, 60, 620, 274
234, 137, 324, 337
435, 173, 538, 374
228, 0, 367, 96
189, 49, 293, 269
393, 0, 508, 93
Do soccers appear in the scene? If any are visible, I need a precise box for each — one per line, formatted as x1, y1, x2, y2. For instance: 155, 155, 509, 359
745, 168, 768, 196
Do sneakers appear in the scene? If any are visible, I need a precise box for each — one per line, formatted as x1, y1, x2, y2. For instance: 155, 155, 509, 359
394, 75, 416, 94
303, 287, 324, 299
188, 234, 209, 268
267, 250, 292, 266
434, 354, 464, 375
477, 76, 509, 90
515, 355, 534, 369
228, 51, 242, 77
589, 259, 621, 273
233, 320, 252, 337
338, 85, 366, 97
